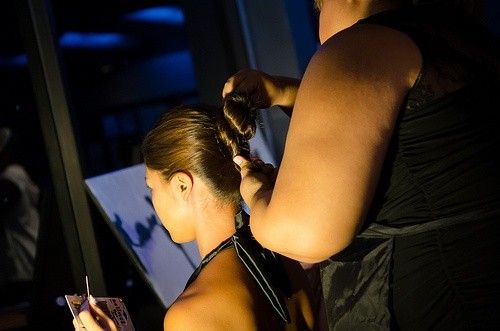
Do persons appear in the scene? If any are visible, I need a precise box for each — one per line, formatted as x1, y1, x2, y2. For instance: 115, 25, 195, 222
72, 94, 320, 331
223, 0, 500, 331
